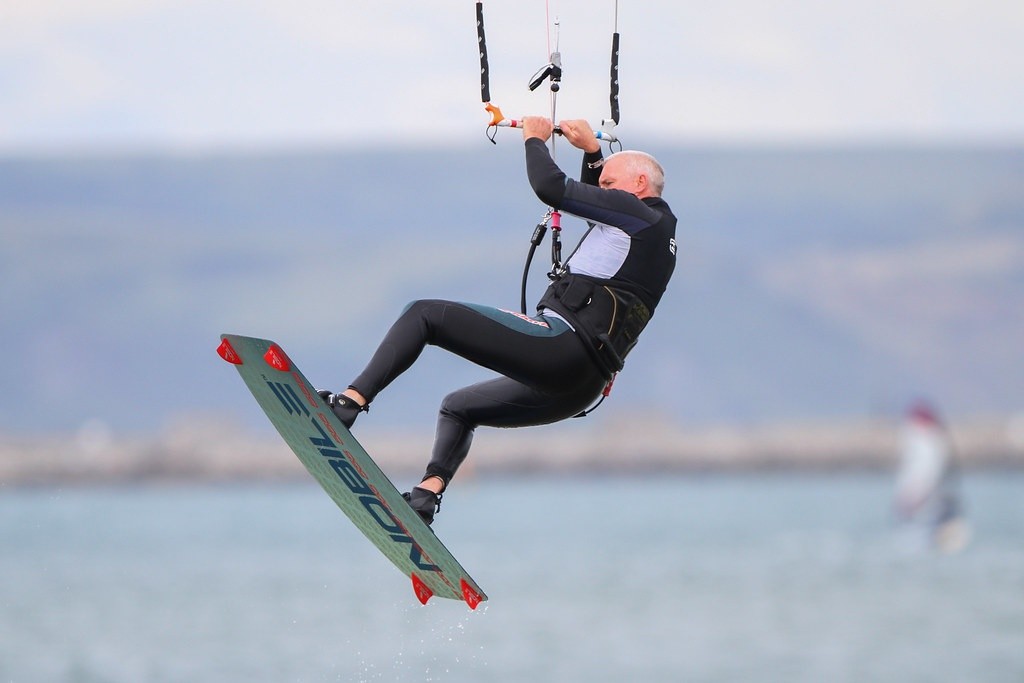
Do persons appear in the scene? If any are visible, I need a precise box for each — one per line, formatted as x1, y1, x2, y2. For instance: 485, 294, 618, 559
896, 406, 961, 539
309, 117, 677, 528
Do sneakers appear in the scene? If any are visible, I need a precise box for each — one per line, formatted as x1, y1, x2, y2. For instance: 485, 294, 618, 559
315, 388, 370, 429
402, 487, 442, 524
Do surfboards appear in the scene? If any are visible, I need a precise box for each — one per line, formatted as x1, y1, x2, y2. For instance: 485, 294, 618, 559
216, 333, 488, 610
